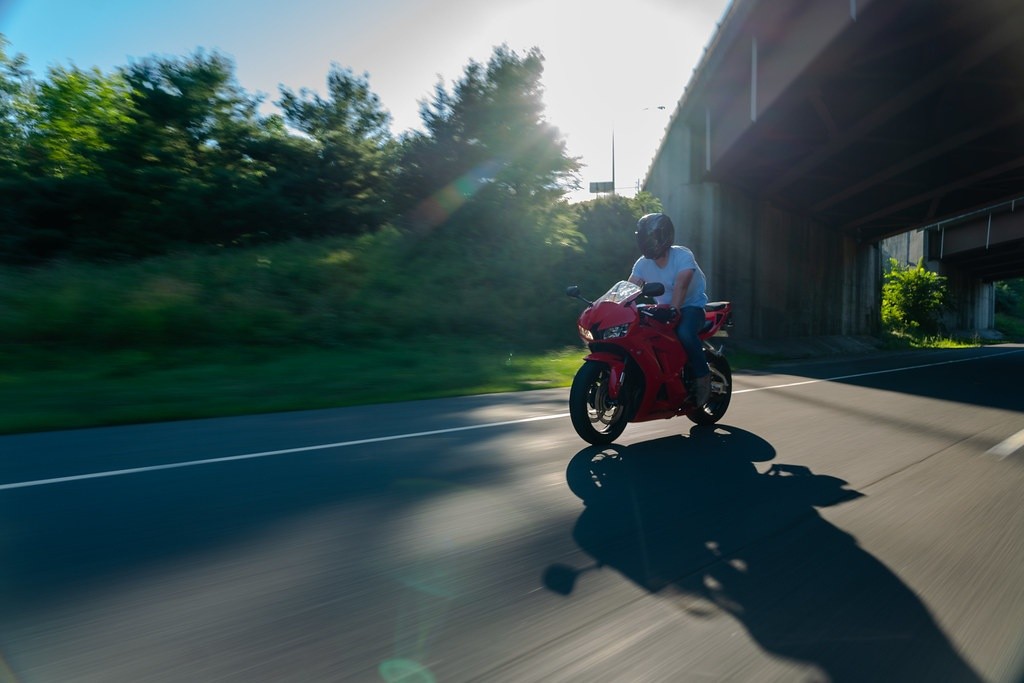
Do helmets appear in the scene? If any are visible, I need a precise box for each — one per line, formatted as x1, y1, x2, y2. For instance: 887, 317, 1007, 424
637, 213, 674, 259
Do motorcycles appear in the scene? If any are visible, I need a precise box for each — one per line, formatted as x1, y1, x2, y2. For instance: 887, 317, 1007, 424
568, 282, 733, 445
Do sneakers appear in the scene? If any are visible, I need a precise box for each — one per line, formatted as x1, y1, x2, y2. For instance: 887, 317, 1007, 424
695, 371, 711, 406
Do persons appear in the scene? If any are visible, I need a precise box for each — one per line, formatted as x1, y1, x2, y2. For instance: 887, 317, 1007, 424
626, 212, 714, 408
